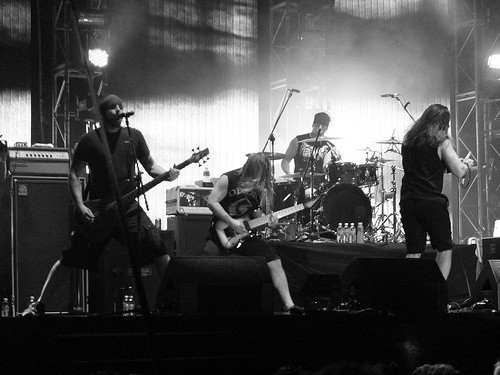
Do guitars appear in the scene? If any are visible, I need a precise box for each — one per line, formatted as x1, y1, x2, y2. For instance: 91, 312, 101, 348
212, 194, 323, 253
72, 145, 209, 242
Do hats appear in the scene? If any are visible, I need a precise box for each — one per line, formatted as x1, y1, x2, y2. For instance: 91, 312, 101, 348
99, 95, 123, 113
314, 112, 330, 126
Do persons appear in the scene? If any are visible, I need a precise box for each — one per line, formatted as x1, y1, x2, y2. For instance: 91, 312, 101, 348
281, 112, 341, 238
399, 104, 474, 280
203, 151, 305, 314
21, 94, 180, 317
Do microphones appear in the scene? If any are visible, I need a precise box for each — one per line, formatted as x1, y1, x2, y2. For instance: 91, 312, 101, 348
380, 93, 400, 97
116, 111, 134, 119
289, 89, 301, 93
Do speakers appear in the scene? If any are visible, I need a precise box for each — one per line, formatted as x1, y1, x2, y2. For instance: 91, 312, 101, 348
170, 253, 275, 315
339, 257, 451, 312
165, 214, 216, 256
0, 175, 82, 316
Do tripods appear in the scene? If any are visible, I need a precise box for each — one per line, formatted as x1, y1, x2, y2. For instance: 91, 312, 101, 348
255, 92, 338, 243
364, 143, 406, 243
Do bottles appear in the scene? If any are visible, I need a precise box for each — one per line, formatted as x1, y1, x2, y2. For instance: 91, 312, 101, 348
2, 298, 9, 317
121, 295, 136, 317
357, 222, 364, 243
349, 223, 356, 243
28, 296, 35, 312
343, 223, 350, 243
337, 223, 344, 243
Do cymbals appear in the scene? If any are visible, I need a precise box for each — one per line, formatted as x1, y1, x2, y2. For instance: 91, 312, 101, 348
246, 152, 286, 160
354, 148, 378, 152
370, 158, 396, 163
296, 136, 343, 143
375, 139, 402, 144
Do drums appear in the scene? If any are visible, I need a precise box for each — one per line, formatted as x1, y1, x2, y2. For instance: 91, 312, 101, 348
270, 159, 380, 239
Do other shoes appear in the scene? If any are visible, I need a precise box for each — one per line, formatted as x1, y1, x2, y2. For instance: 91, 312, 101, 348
22, 301, 45, 316
283, 305, 305, 313
